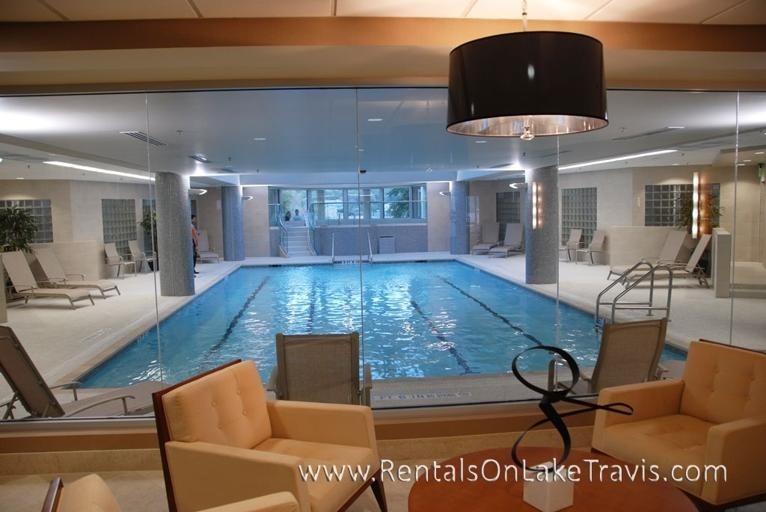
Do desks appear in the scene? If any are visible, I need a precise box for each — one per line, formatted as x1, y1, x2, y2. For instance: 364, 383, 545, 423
408, 447, 699, 512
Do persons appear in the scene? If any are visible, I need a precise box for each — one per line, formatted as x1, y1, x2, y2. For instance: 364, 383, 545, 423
292, 209, 303, 221
285, 210, 292, 221
191, 214, 201, 277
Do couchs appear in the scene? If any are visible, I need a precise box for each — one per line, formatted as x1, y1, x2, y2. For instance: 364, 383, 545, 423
42, 473, 301, 512
151, 357, 388, 512
593, 338, 766, 506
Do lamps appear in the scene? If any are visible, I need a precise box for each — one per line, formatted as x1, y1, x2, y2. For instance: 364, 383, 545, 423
448, 0, 609, 138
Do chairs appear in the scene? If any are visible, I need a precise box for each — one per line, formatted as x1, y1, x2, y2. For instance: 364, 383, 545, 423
490, 221, 525, 257
575, 227, 607, 265
474, 219, 500, 254
192, 228, 217, 263
26, 240, 120, 297
0, 325, 175, 422
101, 243, 135, 277
127, 238, 157, 271
621, 235, 711, 283
273, 333, 373, 420
0, 250, 96, 307
606, 230, 692, 277
557, 315, 669, 398
558, 227, 583, 263
